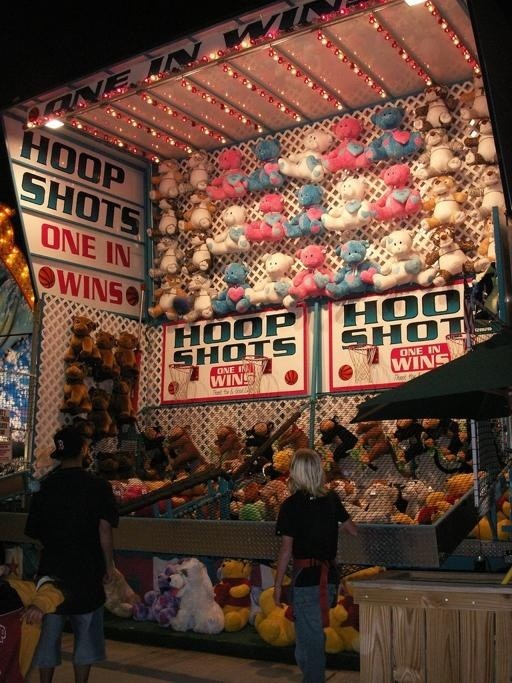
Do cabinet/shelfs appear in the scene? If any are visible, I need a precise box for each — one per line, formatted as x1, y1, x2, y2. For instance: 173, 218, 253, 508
0, 463, 511, 675
347, 571, 512, 683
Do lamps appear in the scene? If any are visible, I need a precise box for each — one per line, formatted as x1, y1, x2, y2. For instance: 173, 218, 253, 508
316, 30, 389, 101
267, 46, 346, 112
141, 91, 227, 144
23, 101, 89, 130
100, 54, 211, 101
66, 116, 161, 164
426, 0, 482, 76
104, 104, 193, 154
367, 12, 433, 86
218, 0, 388, 56
219, 62, 303, 123
180, 79, 266, 134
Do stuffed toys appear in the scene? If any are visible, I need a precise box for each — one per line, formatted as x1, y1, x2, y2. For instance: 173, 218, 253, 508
183, 271, 214, 321
325, 240, 383, 300
206, 149, 248, 200
114, 331, 138, 381
313, 416, 358, 466
82, 388, 113, 435
283, 183, 327, 238
206, 205, 250, 254
149, 237, 185, 282
111, 382, 136, 424
421, 87, 451, 131
333, 473, 478, 524
356, 422, 391, 464
368, 165, 421, 220
320, 179, 371, 232
243, 194, 285, 241
148, 159, 182, 204
182, 230, 211, 275
245, 253, 297, 310
417, 128, 461, 180
63, 315, 101, 362
215, 427, 241, 470
178, 190, 215, 232
245, 422, 272, 469
471, 74, 491, 118
467, 472, 512, 543
132, 564, 179, 627
425, 228, 488, 286
162, 426, 207, 477
287, 245, 336, 301
140, 425, 168, 480
323, 594, 361, 654
60, 365, 91, 413
248, 139, 285, 192
277, 423, 309, 452
372, 231, 436, 291
325, 118, 372, 172
468, 167, 506, 218
108, 478, 212, 520
210, 263, 251, 316
256, 567, 297, 646
103, 560, 140, 618
421, 176, 468, 231
472, 218, 496, 273
178, 150, 215, 193
166, 557, 224, 635
391, 418, 430, 464
365, 108, 420, 163
215, 479, 290, 521
146, 197, 178, 237
426, 420, 466, 473
93, 330, 120, 383
278, 133, 331, 182
463, 120, 499, 166
213, 560, 253, 632
147, 276, 186, 321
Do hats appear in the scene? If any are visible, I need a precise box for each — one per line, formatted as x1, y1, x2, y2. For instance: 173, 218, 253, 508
50, 427, 83, 461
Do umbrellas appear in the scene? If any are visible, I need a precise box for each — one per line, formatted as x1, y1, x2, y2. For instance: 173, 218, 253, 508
348, 329, 512, 467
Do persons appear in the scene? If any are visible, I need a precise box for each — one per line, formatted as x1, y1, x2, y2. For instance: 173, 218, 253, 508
273, 449, 357, 683
25, 426, 120, 683
0, 546, 64, 683
